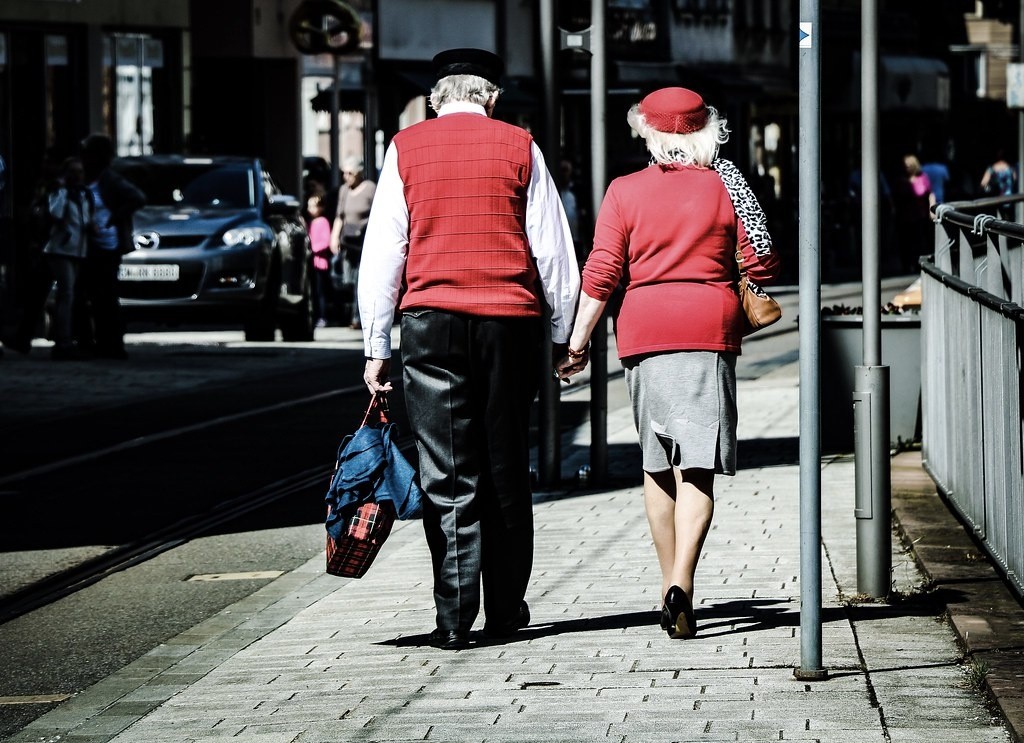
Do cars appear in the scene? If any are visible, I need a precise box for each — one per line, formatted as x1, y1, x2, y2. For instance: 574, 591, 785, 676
110, 153, 317, 344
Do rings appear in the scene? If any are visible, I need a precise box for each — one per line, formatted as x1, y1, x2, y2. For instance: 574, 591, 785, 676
366, 380, 371, 386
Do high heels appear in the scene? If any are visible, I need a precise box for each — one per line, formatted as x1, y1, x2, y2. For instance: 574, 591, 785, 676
660, 584, 697, 638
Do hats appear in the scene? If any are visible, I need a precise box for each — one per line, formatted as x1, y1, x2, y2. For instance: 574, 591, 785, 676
432, 48, 502, 88
638, 87, 711, 134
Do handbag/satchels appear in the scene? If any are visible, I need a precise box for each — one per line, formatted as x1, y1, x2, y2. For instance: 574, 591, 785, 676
326, 389, 396, 579
731, 244, 782, 337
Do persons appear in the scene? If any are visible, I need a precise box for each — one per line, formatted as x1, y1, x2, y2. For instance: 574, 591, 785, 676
357, 49, 593, 651
0, 133, 1020, 361
557, 88, 773, 638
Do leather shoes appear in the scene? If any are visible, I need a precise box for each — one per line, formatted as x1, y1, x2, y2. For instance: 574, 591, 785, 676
429, 628, 472, 650
482, 601, 530, 644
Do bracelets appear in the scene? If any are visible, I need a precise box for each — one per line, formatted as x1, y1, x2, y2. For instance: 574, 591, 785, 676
567, 337, 593, 358
